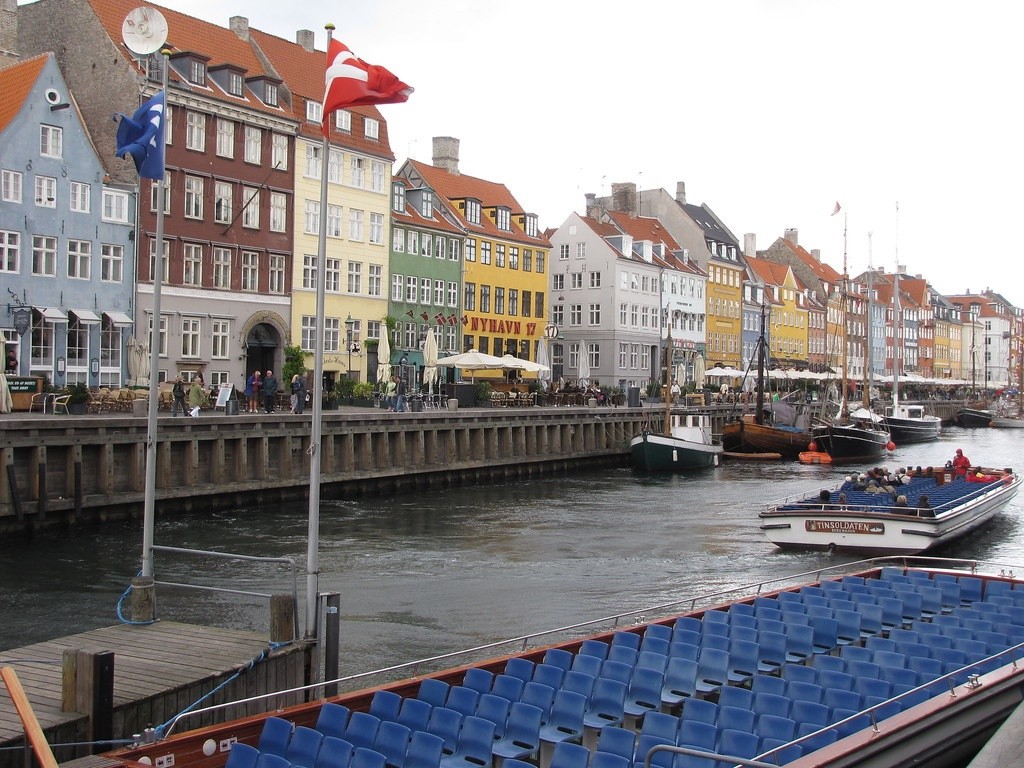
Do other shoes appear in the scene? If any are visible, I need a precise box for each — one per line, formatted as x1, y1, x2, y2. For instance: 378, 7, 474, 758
391, 410, 393, 411
399, 410, 404, 412
295, 412, 297, 414
386, 409, 390, 412
265, 411, 268, 414
394, 410, 397, 412
254, 410, 258, 414
270, 411, 274, 414
250, 409, 252, 412
290, 411, 292, 413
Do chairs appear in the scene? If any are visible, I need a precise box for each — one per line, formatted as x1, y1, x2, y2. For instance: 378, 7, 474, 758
778, 473, 1006, 515
403, 392, 449, 411
224, 566, 1024, 768
85, 387, 211, 415
29, 392, 72, 415
488, 391, 626, 407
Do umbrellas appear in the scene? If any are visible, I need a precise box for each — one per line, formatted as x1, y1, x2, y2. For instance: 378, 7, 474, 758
0, 329, 13, 413
126, 334, 151, 387
537, 336, 550, 392
422, 327, 437, 406
376, 322, 392, 384
706, 359, 1019, 392
437, 349, 551, 383
577, 339, 590, 406
678, 354, 705, 392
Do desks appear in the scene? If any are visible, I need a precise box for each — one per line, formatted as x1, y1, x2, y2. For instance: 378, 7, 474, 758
42, 393, 61, 415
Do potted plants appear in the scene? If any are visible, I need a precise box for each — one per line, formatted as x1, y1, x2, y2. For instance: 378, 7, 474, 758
611, 385, 626, 406
471, 380, 491, 407
645, 378, 662, 403
335, 376, 376, 408
379, 381, 388, 408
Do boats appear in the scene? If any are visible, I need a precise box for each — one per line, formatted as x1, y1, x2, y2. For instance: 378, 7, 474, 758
58, 553, 1024, 768
759, 464, 1024, 557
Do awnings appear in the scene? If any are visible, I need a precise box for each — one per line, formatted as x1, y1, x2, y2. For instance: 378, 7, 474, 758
36, 307, 135, 328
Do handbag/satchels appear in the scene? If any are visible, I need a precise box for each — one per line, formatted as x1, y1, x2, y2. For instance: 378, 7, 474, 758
174, 391, 183, 398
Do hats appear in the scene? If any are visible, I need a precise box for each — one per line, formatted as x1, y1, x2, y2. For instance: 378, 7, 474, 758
859, 474, 867, 479
513, 382, 517, 385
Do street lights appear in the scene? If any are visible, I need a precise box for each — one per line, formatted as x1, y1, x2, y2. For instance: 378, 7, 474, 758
345, 311, 355, 381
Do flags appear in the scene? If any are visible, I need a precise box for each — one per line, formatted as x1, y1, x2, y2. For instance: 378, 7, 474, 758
322, 37, 414, 139
830, 201, 843, 217
405, 309, 468, 326
113, 92, 164, 180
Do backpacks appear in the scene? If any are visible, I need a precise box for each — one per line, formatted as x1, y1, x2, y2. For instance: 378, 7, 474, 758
294, 378, 302, 391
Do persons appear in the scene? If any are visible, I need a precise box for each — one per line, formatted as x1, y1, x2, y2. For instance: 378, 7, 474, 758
841, 466, 934, 494
945, 448, 1012, 482
807, 490, 854, 511
172, 370, 308, 417
670, 378, 806, 407
891, 495, 934, 517
872, 384, 1022, 419
6, 350, 18, 370
507, 374, 602, 407
386, 376, 405, 412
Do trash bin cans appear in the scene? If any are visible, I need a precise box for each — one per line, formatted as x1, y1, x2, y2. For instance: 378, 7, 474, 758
226, 400, 239, 415
588, 398, 597, 408
448, 398, 458, 411
884, 392, 891, 401
132, 399, 147, 417
694, 389, 711, 405
627, 387, 640, 406
411, 396, 423, 412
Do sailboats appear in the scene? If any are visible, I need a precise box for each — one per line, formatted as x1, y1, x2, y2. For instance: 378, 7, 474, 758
951, 312, 995, 428
989, 317, 1024, 429
720, 292, 816, 458
811, 211, 890, 463
630, 323, 724, 474
860, 201, 941, 444
